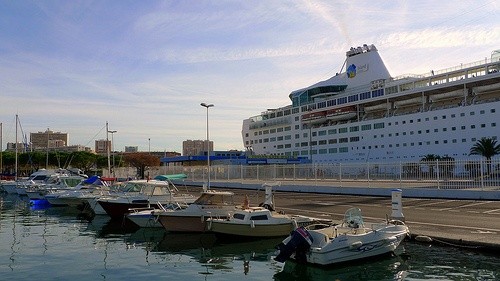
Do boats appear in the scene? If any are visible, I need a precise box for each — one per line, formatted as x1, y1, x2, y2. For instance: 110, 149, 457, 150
270, 207, 411, 274
0, 113, 254, 235
428, 87, 469, 104
471, 82, 500, 97
301, 110, 329, 129
363, 101, 393, 114
205, 193, 339, 243
393, 95, 428, 110
325, 105, 365, 123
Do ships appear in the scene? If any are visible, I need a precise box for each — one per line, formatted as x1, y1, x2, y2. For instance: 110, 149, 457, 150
241, 43, 500, 180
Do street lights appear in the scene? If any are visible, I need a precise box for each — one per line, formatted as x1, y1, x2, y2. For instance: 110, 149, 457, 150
108, 130, 117, 177
200, 102, 215, 190
